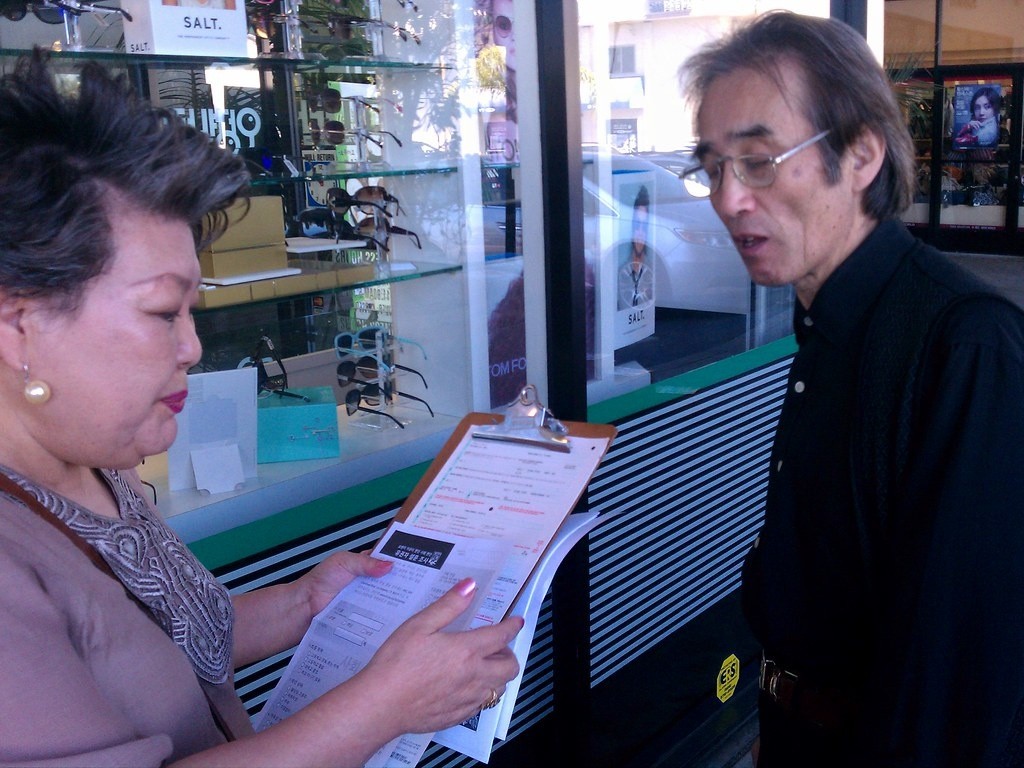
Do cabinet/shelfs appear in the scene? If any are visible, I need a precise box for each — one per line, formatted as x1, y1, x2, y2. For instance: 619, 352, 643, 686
0, 48, 464, 314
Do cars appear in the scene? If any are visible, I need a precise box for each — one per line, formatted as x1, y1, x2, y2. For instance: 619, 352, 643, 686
418, 151, 749, 317
375, 140, 720, 265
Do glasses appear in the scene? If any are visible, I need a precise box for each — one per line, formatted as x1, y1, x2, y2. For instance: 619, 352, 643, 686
242, 0, 435, 432
678, 125, 835, 199
0, 0, 134, 25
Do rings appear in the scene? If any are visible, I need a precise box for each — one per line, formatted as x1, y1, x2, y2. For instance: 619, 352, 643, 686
481, 689, 501, 710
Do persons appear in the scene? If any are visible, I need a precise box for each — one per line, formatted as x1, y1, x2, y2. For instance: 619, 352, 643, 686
617, 185, 654, 311
476, 0, 520, 162
955, 87, 1000, 147
677, 12, 1024, 768
0, 55, 523, 768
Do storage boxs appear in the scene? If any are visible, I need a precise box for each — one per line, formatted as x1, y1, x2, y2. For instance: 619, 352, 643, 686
257, 386, 341, 464
198, 196, 288, 280
120, 0, 248, 57
